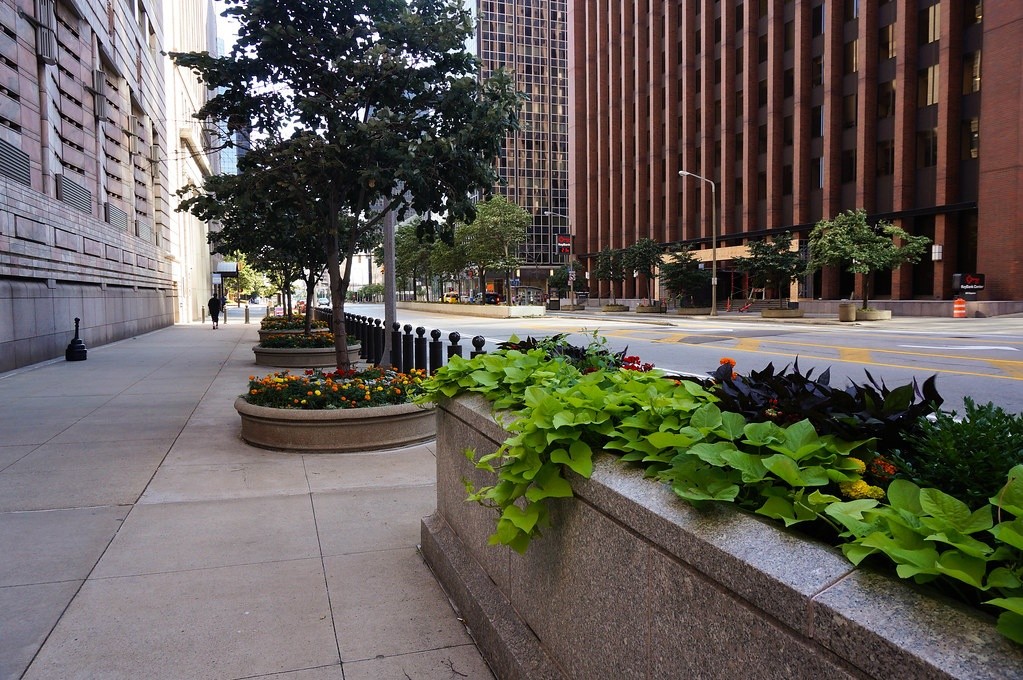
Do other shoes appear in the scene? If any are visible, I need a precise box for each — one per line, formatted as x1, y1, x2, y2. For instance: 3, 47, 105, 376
213, 325, 219, 329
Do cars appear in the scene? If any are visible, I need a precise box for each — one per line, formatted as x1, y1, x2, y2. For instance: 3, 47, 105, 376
475, 292, 500, 304
460, 296, 469, 302
439, 292, 459, 304
318, 298, 330, 306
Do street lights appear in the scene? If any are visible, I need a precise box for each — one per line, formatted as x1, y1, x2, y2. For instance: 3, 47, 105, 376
544, 211, 576, 311
679, 171, 719, 318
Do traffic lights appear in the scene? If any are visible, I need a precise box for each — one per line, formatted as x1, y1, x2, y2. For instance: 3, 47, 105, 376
381, 267, 385, 274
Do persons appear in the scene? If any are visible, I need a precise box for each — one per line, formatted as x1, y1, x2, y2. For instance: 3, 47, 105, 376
208, 293, 226, 329
512, 293, 550, 307
